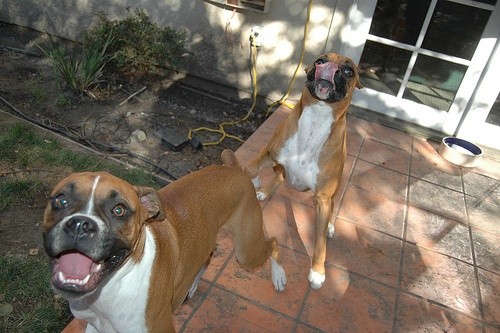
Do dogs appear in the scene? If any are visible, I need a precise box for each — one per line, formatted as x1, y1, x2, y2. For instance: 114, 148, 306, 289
43, 148, 287, 333
245, 54, 365, 290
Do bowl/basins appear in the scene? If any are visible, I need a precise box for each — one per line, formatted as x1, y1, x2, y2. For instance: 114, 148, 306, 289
438, 137, 483, 167
